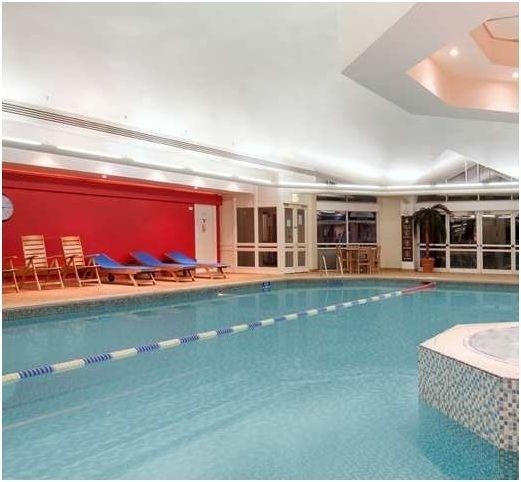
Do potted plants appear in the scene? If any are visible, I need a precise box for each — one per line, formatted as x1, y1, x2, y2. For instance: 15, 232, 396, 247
408, 205, 455, 273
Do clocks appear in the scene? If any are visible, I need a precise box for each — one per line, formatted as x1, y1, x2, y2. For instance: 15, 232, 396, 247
2, 194, 14, 222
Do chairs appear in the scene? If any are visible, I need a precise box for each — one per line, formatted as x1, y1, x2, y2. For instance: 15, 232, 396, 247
2, 233, 231, 294
337, 245, 382, 275
